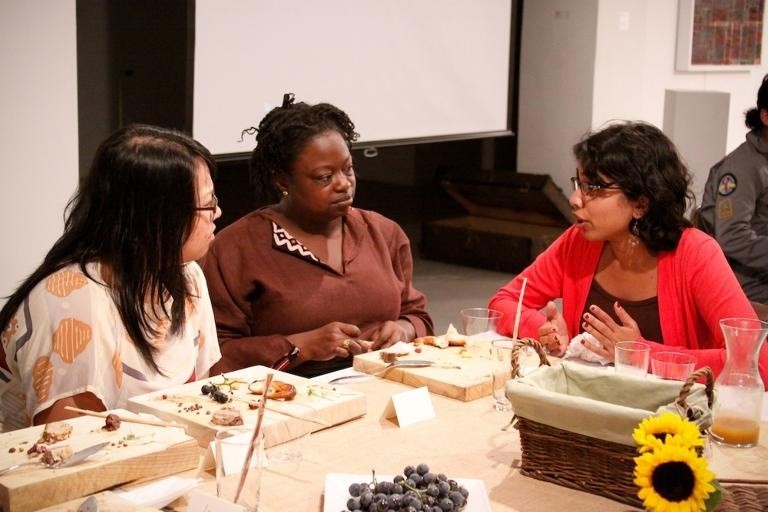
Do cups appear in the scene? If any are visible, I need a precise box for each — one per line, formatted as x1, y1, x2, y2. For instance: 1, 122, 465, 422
213, 423, 267, 512
614, 340, 651, 377
459, 306, 507, 356
649, 351, 698, 383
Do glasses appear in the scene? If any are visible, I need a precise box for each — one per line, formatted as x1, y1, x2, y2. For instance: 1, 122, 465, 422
567, 173, 624, 195
191, 189, 221, 218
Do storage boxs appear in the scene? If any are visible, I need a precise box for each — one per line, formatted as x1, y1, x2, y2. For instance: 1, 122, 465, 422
420, 163, 576, 275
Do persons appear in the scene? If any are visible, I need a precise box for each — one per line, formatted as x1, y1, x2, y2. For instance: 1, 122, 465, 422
697, 73, 768, 328
193, 92, 435, 379
487, 120, 767, 392
1, 121, 223, 435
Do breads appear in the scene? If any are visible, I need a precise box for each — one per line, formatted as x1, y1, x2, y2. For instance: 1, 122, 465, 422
44, 421, 73, 443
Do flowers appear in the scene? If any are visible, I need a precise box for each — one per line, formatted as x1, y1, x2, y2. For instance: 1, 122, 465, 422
631, 406, 722, 512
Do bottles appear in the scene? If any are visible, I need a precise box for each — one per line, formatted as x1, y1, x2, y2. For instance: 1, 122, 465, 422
709, 318, 768, 449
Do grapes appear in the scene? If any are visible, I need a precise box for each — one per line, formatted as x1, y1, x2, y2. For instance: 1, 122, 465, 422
201, 385, 229, 403
346, 464, 468, 512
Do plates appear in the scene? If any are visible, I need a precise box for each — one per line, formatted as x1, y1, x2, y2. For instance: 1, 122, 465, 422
323, 471, 494, 511
